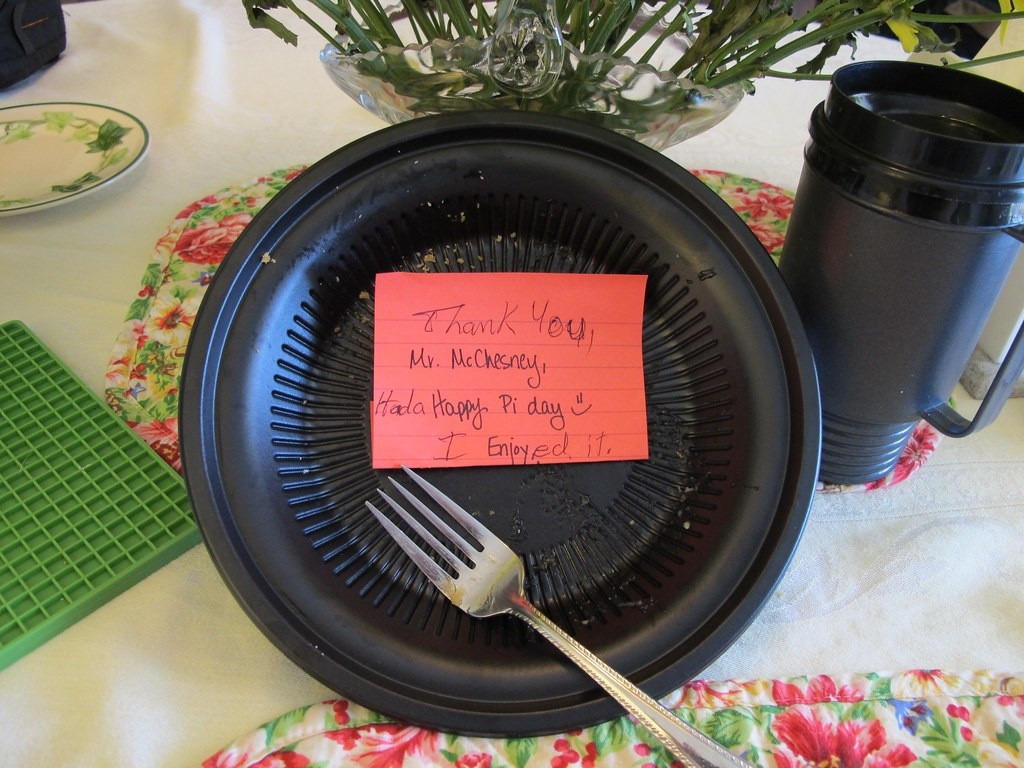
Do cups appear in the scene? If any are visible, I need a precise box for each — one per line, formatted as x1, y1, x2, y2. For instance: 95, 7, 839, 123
778, 60, 1024, 485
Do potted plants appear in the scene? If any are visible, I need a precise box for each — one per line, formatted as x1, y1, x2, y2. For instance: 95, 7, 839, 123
242, 0, 1024, 153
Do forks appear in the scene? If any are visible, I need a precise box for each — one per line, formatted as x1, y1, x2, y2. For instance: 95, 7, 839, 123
365, 463, 755, 768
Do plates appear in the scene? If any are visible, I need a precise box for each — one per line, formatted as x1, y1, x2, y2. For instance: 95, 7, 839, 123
176, 110, 823, 739
0, 102, 151, 217
